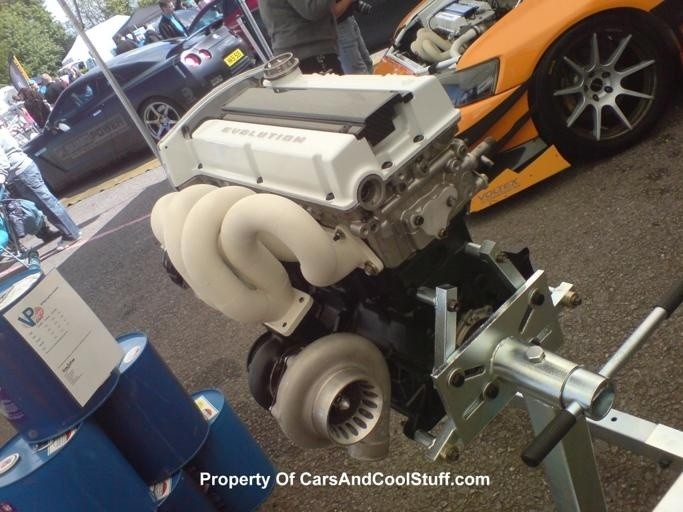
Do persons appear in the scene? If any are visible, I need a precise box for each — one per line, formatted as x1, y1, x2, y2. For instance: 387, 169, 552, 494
256, 0, 342, 75
331, 0, 375, 75
179, 0, 200, 12
111, 34, 137, 55
156, 0, 190, 38
0, 136, 81, 255
65, 64, 93, 104
141, 23, 160, 44
0, 186, 60, 245
0, 71, 69, 147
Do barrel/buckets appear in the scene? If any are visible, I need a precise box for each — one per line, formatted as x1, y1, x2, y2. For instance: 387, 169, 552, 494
2, 246, 277, 510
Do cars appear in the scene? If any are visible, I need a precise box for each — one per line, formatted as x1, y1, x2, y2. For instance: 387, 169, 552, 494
187, 0, 272, 60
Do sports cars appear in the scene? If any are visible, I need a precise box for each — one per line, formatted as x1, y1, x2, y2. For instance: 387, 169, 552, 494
22, 19, 258, 198
371, 1, 680, 219
